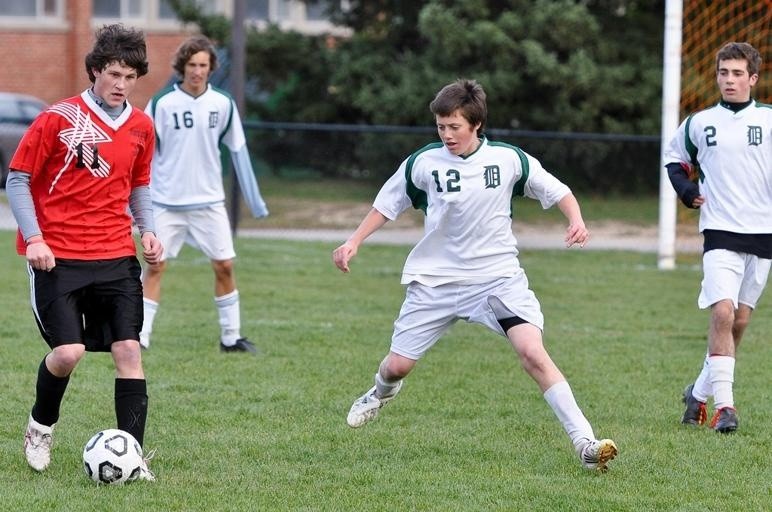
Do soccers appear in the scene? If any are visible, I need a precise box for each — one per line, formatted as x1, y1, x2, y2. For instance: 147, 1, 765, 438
81, 428, 144, 489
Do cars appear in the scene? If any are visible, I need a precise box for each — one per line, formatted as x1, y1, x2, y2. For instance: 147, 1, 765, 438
0, 93, 51, 188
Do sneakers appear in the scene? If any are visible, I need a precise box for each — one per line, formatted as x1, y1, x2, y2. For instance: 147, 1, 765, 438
139, 331, 150, 349
680, 383, 707, 425
579, 437, 617, 470
140, 458, 156, 481
709, 406, 738, 433
219, 336, 256, 352
346, 379, 403, 428
24, 411, 56, 471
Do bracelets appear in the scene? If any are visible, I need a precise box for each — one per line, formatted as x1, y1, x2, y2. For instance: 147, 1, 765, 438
27, 239, 46, 246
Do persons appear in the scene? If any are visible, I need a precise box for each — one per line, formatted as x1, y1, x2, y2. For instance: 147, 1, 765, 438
139, 34, 270, 355
332, 80, 619, 475
662, 42, 772, 437
5, 20, 165, 481
